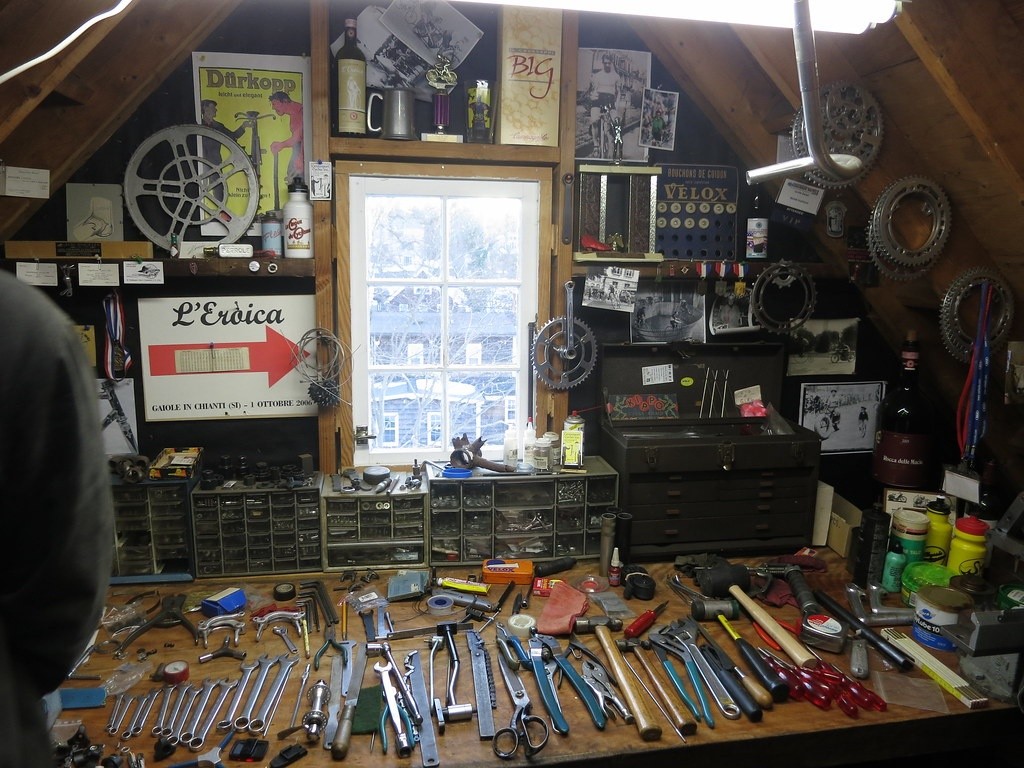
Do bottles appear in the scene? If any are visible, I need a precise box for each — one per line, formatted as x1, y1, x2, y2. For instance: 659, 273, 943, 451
502, 409, 586, 474
739, 180, 769, 264
881, 540, 907, 592
853, 496, 892, 590
889, 509, 929, 564
332, 13, 368, 138
875, 330, 934, 491
947, 514, 992, 579
203, 244, 254, 257
921, 494, 952, 567
281, 177, 316, 259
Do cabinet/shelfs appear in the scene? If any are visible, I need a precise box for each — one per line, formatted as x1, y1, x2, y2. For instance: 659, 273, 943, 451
596, 341, 822, 564
112, 455, 619, 585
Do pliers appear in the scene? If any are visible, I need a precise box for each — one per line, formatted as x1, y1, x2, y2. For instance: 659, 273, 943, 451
495, 620, 533, 670
557, 633, 619, 691
529, 632, 606, 733
115, 593, 199, 649
647, 633, 714, 729
744, 608, 799, 651
580, 659, 635, 724
314, 622, 347, 669
379, 689, 415, 752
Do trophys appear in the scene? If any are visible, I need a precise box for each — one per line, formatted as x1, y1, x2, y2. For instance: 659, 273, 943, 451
420, 54, 464, 144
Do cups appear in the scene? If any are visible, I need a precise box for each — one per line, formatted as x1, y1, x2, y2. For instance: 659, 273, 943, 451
367, 87, 423, 140
464, 80, 498, 144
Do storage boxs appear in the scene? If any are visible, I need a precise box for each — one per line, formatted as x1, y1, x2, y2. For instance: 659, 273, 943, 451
148, 447, 204, 481
812, 480, 863, 558
482, 558, 534, 584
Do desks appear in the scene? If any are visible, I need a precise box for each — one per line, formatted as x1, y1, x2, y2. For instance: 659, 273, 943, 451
58, 543, 1023, 767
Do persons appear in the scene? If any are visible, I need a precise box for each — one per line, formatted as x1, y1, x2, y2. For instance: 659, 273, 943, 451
652, 110, 663, 142
0, 264, 114, 767
588, 53, 621, 159
858, 406, 869, 432
827, 389, 840, 415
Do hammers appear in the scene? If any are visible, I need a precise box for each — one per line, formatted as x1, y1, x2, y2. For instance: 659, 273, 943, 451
691, 599, 789, 702
616, 640, 698, 737
703, 564, 819, 668
574, 616, 662, 740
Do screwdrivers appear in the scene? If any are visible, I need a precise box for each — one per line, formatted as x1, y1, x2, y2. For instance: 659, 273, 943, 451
625, 598, 670, 639
761, 643, 887, 716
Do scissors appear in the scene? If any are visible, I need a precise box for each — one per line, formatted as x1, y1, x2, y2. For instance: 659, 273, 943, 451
492, 650, 549, 759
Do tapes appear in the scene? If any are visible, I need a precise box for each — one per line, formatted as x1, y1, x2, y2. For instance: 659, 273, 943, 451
273, 582, 297, 600
425, 596, 454, 615
507, 613, 536, 634
164, 660, 188, 684
